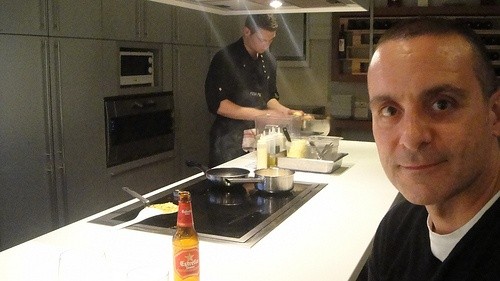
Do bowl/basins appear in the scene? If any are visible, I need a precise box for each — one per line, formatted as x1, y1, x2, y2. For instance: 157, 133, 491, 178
300, 113, 331, 136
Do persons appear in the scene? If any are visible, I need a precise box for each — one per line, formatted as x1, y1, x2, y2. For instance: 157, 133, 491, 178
204, 13, 310, 168
356, 16, 500, 281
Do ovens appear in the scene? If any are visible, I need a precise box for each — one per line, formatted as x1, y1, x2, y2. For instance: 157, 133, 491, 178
104, 91, 175, 168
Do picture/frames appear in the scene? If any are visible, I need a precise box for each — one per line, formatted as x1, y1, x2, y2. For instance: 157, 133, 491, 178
268, 13, 310, 68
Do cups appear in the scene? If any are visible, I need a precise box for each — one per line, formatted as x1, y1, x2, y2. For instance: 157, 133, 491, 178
285, 139, 309, 158
307, 135, 343, 161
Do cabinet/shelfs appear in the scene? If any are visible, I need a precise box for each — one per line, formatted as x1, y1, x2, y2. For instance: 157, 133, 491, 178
0, 33, 106, 253
0, 0, 234, 47
332, 12, 500, 84
176, 43, 223, 178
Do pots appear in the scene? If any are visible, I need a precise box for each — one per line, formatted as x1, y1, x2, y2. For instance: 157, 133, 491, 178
225, 167, 295, 194
184, 159, 250, 189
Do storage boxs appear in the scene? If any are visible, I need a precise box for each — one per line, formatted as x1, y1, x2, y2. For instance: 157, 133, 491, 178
275, 133, 349, 174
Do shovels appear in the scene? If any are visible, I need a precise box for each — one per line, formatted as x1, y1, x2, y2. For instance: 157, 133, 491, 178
121, 187, 178, 212
112, 207, 164, 231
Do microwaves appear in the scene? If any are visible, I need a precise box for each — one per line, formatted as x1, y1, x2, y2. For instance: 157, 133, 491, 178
120, 51, 154, 89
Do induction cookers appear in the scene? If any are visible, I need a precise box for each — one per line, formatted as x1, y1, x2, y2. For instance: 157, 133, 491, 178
87, 174, 328, 248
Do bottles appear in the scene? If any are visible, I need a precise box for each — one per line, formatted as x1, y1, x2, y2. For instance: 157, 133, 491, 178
256, 126, 287, 170
338, 23, 346, 58
172, 191, 200, 281
